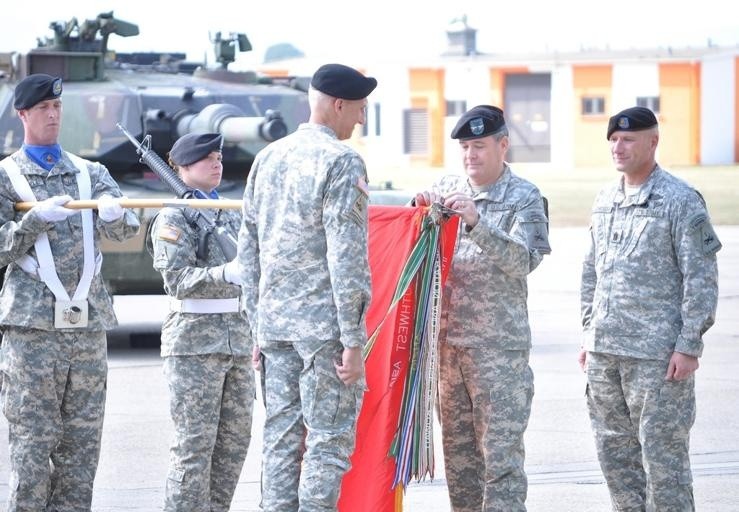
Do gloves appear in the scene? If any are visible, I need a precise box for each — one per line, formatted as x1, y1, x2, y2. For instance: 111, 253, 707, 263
224, 256, 243, 285
97, 194, 127, 222
37, 194, 81, 221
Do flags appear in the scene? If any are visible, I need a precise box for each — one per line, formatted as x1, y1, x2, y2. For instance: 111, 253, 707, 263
338, 204, 459, 511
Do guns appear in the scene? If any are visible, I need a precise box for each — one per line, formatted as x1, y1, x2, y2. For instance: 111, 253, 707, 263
116, 124, 238, 262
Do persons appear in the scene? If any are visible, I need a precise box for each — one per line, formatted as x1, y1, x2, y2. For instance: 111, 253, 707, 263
151, 133, 256, 512
404, 105, 552, 512
236, 63, 378, 512
579, 108, 723, 512
0, 73, 142, 512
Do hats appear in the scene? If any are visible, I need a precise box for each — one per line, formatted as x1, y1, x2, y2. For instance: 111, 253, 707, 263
607, 107, 657, 140
311, 64, 377, 99
170, 133, 222, 165
450, 105, 505, 140
14, 74, 62, 110
